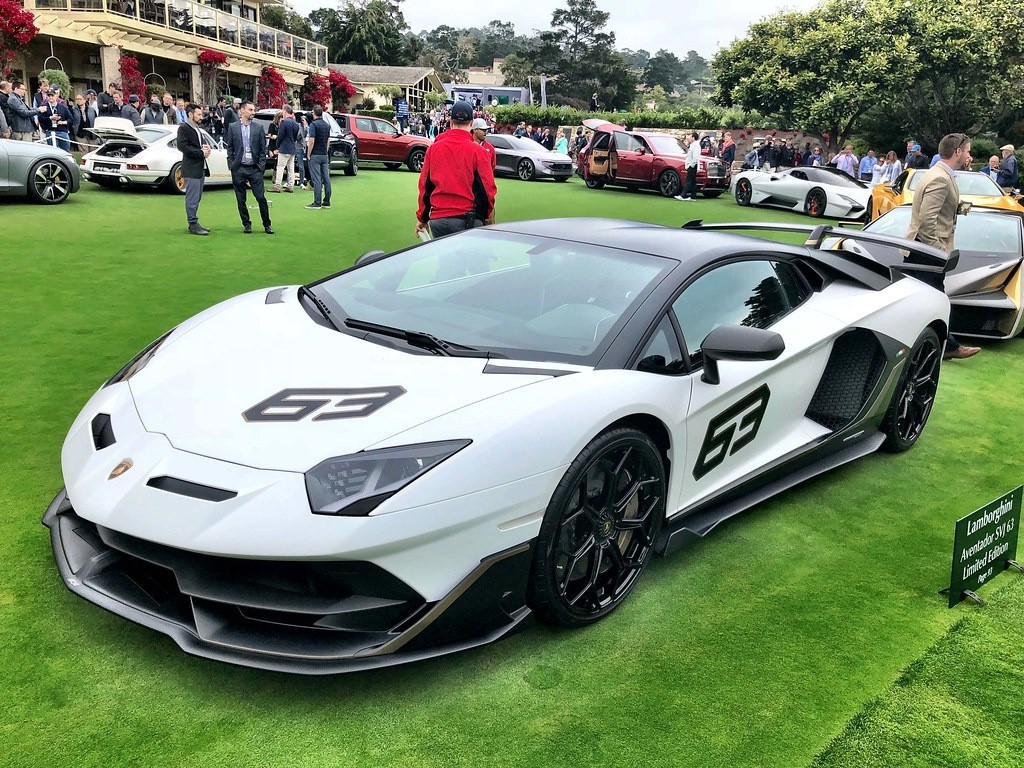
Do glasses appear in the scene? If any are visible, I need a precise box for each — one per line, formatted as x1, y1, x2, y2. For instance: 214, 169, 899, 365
879, 160, 884, 162
815, 150, 819, 151
956, 134, 968, 152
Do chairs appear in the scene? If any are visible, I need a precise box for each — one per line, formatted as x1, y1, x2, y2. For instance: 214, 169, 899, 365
78, 0, 310, 64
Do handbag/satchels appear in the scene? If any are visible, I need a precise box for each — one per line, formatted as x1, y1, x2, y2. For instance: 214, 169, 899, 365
740, 164, 750, 172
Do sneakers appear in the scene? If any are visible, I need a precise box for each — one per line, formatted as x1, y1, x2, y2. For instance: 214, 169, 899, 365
942, 344, 981, 360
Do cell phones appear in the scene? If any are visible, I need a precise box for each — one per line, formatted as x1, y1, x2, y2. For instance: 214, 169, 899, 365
58, 114, 62, 116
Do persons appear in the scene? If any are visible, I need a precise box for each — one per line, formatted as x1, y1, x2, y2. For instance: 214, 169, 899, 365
674, 127, 1019, 209
900, 133, 981, 360
415, 104, 497, 239
406, 102, 594, 162
177, 103, 211, 235
226, 101, 275, 234
305, 105, 331, 210
0, 79, 321, 193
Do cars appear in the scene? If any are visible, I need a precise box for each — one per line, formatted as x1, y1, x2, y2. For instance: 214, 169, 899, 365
485, 134, 573, 182
577, 118, 730, 198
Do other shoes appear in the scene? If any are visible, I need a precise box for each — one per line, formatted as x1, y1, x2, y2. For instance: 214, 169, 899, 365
304, 202, 330, 209
266, 181, 323, 193
674, 195, 696, 201
244, 226, 251, 233
190, 223, 209, 235
266, 226, 274, 234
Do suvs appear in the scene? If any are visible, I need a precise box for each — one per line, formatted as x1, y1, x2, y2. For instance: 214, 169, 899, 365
258, 109, 358, 177
332, 113, 432, 173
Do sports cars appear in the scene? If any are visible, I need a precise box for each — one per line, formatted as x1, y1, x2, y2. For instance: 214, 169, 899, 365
730, 165, 868, 218
0, 138, 80, 204
80, 115, 234, 192
43, 217, 950, 677
839, 167, 1024, 342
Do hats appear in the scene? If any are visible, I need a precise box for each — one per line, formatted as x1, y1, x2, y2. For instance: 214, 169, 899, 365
470, 118, 492, 129
86, 89, 97, 96
451, 102, 473, 121
234, 98, 242, 103
209, 105, 217, 112
46, 89, 55, 95
753, 143, 760, 147
109, 83, 122, 91
129, 94, 139, 101
50, 84, 59, 90
999, 144, 1014, 151
911, 145, 921, 151
152, 99, 161, 105
